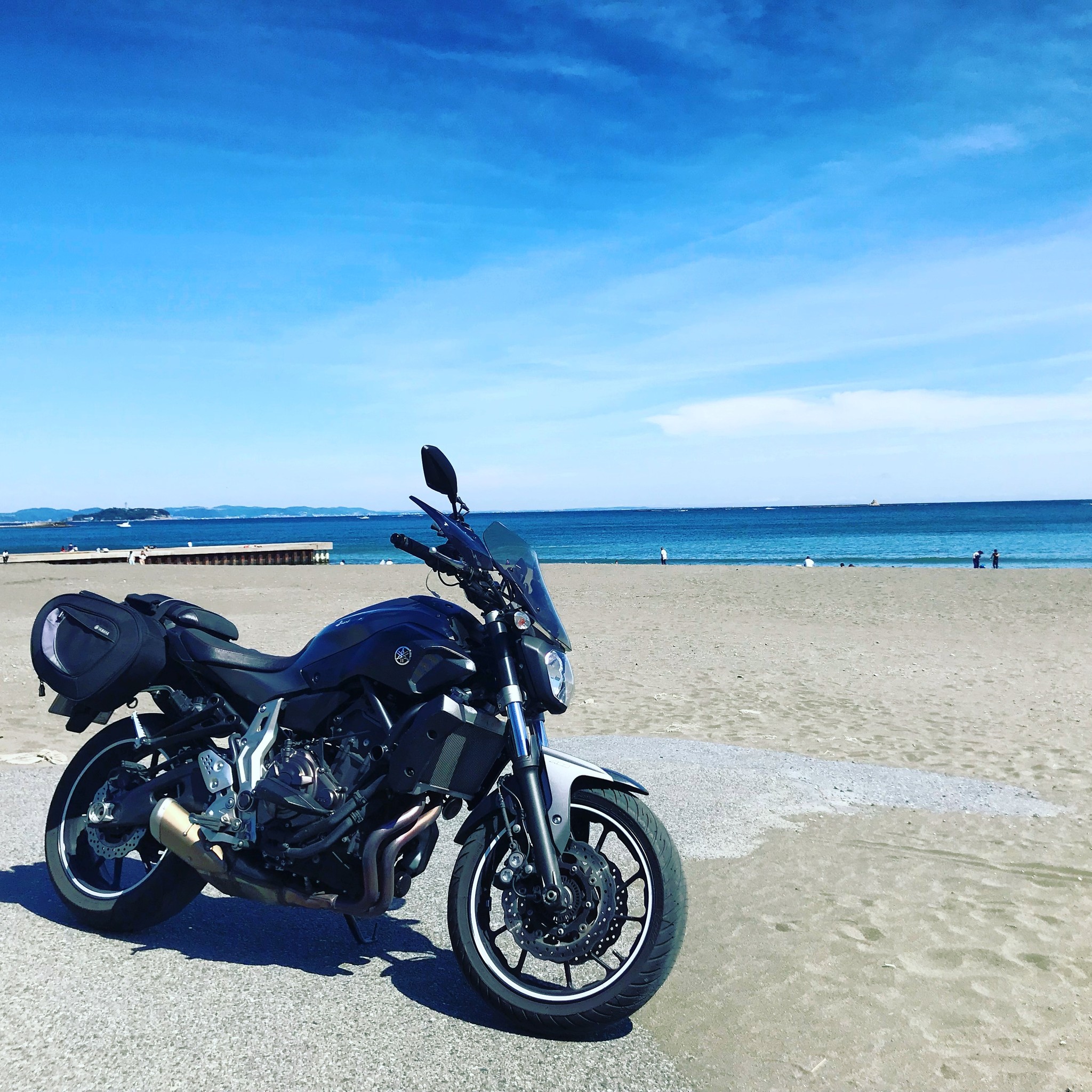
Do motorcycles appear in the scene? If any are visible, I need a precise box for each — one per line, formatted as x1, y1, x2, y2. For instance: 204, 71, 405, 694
28, 446, 689, 1038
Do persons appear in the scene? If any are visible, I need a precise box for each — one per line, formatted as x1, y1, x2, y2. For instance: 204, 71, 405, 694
661, 547, 667, 564
991, 549, 999, 569
803, 556, 813, 567
187, 541, 192, 547
61, 543, 78, 552
505, 558, 510, 565
615, 561, 618, 564
380, 560, 385, 565
3, 550, 8, 564
386, 558, 393, 565
973, 550, 984, 568
849, 564, 854, 567
139, 551, 148, 565
340, 559, 345, 565
127, 551, 135, 565
153, 545, 155, 549
840, 563, 844, 567
148, 545, 150, 549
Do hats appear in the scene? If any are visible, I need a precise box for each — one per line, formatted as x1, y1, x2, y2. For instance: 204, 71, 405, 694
993, 549, 997, 552
